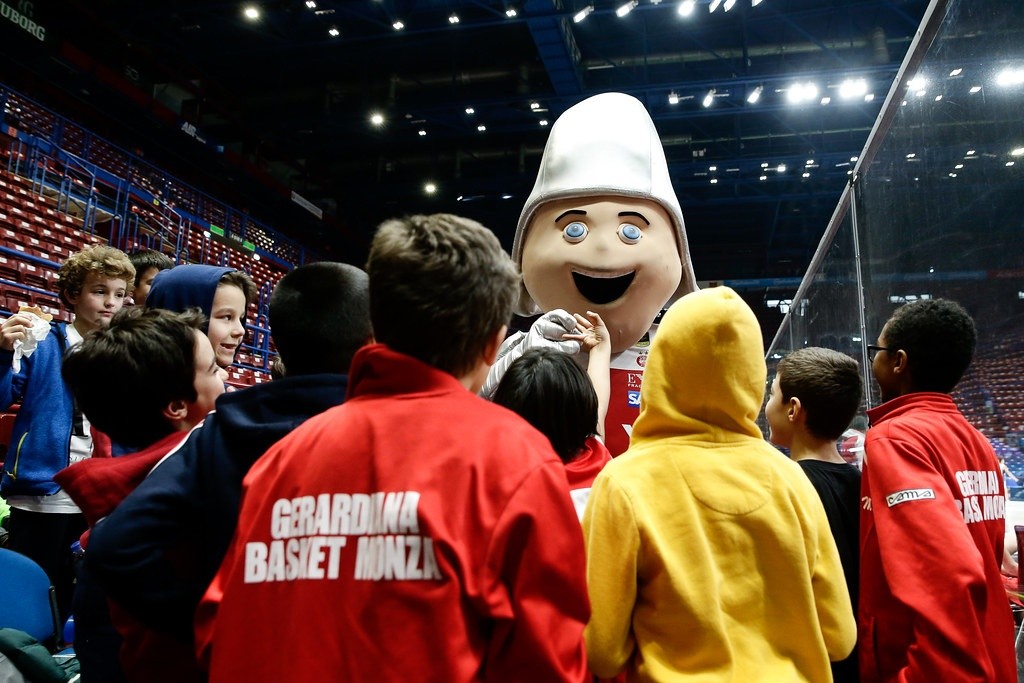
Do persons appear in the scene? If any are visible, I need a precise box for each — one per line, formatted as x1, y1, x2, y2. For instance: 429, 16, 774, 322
478, 92, 699, 459
0, 212, 1024, 683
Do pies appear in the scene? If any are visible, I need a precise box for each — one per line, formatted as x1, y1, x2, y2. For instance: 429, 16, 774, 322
19, 306, 53, 323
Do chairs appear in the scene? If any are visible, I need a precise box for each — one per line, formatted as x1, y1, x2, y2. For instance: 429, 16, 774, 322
947, 332, 1024, 500
0, 85, 307, 661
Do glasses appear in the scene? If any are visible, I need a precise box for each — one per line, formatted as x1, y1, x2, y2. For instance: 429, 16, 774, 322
867, 344, 899, 363
